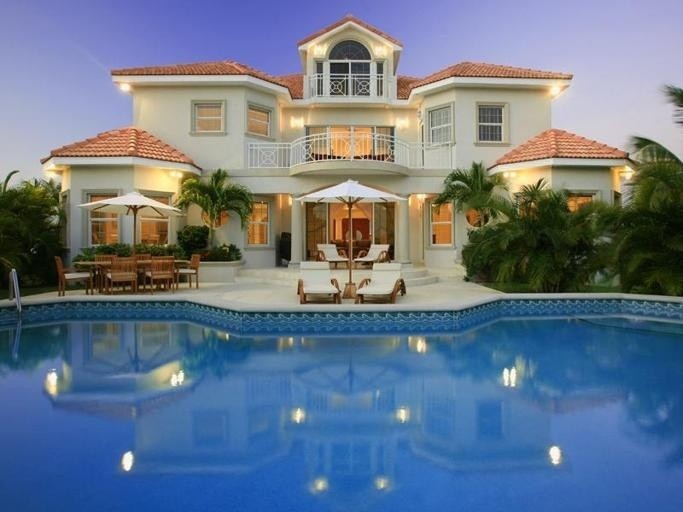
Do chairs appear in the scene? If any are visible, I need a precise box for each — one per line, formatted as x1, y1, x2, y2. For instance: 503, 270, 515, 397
54, 253, 201, 296
297, 239, 406, 305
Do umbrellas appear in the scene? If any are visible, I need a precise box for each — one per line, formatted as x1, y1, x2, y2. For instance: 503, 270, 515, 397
73, 321, 189, 382
75, 192, 183, 296
294, 179, 410, 285
292, 338, 412, 398
